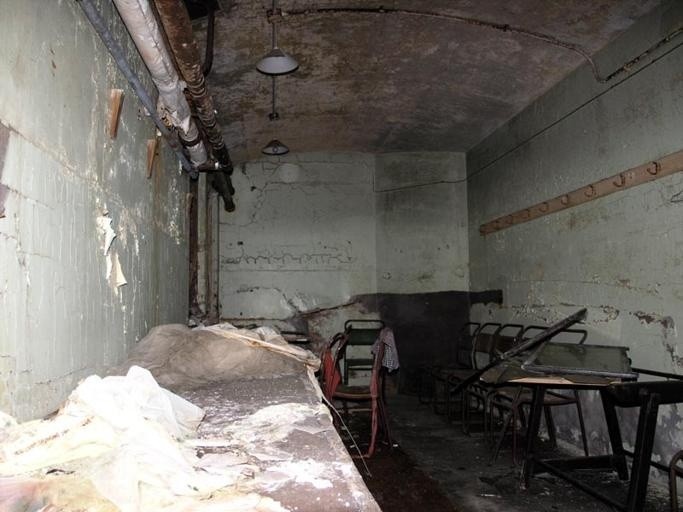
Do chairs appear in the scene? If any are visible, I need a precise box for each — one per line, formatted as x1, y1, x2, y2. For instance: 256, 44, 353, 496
419, 321, 594, 475
317, 316, 393, 459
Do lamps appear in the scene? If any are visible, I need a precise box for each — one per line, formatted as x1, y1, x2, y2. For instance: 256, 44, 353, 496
261, 112, 288, 155
257, 8, 299, 77
